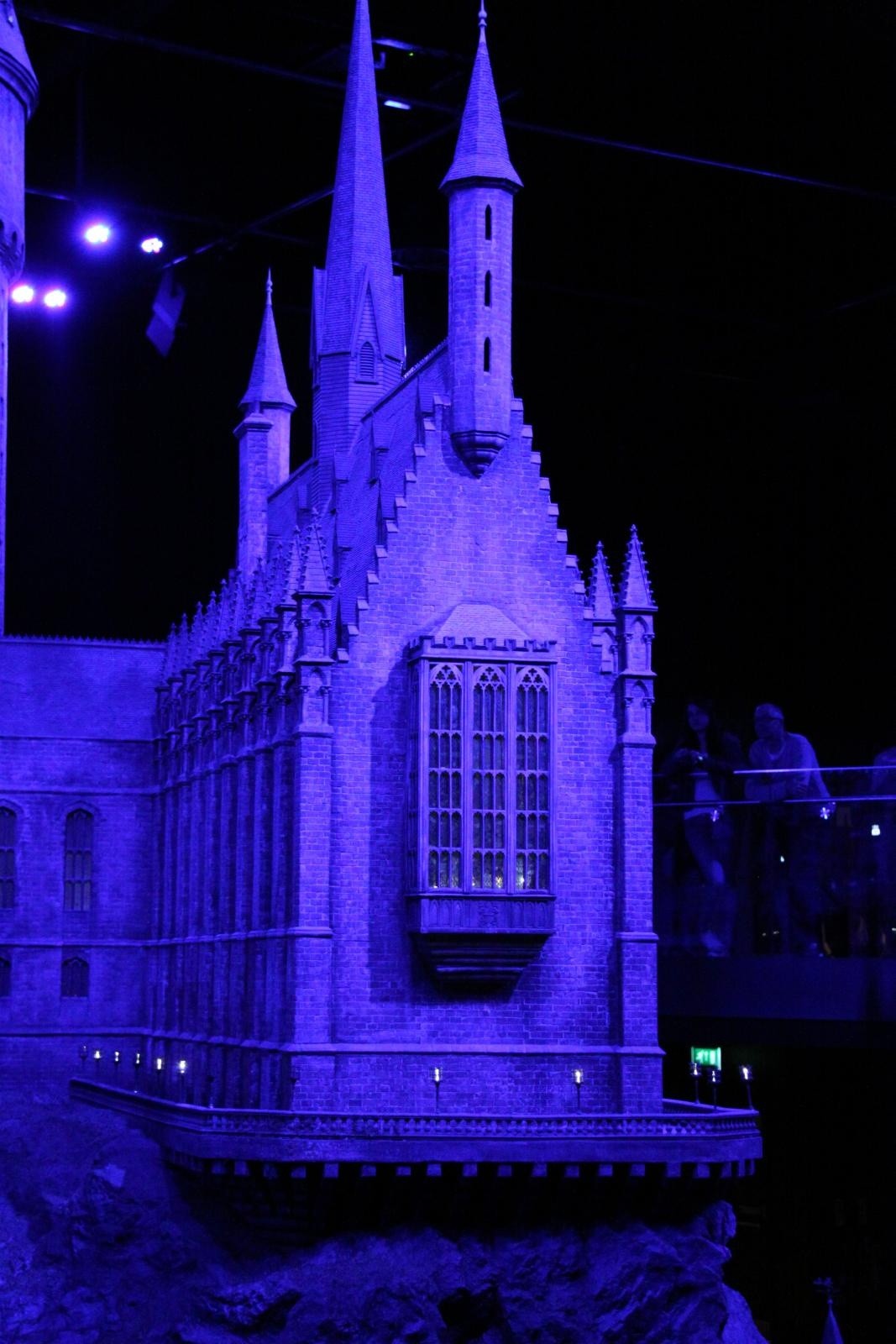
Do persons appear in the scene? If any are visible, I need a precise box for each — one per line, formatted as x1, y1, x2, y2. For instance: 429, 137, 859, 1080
667, 700, 734, 941
745, 704, 828, 951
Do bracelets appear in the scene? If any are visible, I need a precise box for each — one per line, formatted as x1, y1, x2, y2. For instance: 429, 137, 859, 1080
698, 754, 703, 761
